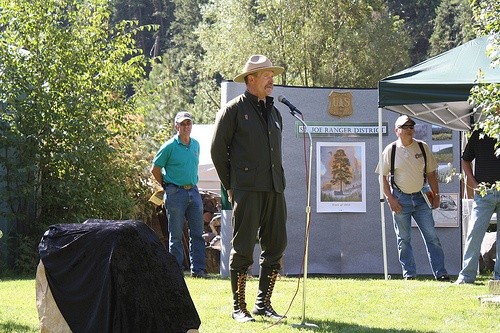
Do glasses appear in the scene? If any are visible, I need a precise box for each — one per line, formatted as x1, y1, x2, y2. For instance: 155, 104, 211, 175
399, 125, 414, 129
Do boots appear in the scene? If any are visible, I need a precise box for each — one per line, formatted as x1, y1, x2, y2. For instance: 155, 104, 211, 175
252, 265, 287, 318
230, 269, 255, 322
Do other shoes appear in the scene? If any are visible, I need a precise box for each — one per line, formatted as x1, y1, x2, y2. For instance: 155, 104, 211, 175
455, 279, 468, 284
437, 274, 451, 281
193, 271, 212, 279
407, 276, 416, 280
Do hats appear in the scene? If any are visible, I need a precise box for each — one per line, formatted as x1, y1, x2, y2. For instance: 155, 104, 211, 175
233, 55, 285, 83
395, 115, 416, 129
175, 112, 192, 123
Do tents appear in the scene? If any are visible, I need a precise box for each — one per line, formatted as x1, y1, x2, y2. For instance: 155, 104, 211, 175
377, 30, 500, 281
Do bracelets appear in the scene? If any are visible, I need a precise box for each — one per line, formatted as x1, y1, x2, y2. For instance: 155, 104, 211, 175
434, 192, 441, 196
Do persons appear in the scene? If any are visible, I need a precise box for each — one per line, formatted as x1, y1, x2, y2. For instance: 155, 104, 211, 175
454, 103, 500, 284
374, 115, 452, 282
151, 111, 214, 280
211, 54, 289, 324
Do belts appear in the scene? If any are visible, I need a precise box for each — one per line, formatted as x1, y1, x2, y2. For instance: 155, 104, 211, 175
173, 183, 196, 189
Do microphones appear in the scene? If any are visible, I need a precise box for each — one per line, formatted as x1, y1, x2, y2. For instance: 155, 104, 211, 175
278, 95, 302, 115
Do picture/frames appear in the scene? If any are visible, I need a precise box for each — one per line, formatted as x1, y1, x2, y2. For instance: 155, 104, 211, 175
412, 192, 459, 228
